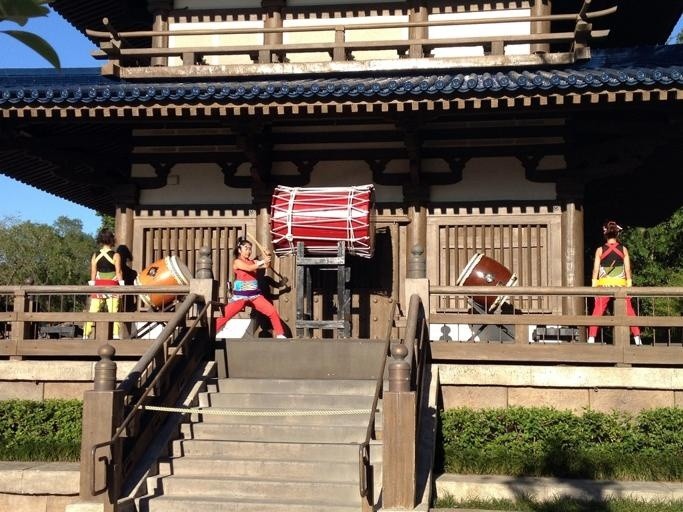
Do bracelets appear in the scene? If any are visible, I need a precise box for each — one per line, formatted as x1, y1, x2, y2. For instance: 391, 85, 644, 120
256, 261, 265, 267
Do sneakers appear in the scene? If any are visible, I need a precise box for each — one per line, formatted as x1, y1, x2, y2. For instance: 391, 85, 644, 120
588, 336, 642, 346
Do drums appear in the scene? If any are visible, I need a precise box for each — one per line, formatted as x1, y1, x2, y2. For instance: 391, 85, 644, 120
268, 184, 376, 259
133, 255, 193, 311
456, 253, 518, 314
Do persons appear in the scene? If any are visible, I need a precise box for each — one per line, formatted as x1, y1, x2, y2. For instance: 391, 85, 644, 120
587, 221, 643, 346
214, 240, 287, 339
83, 228, 126, 340
